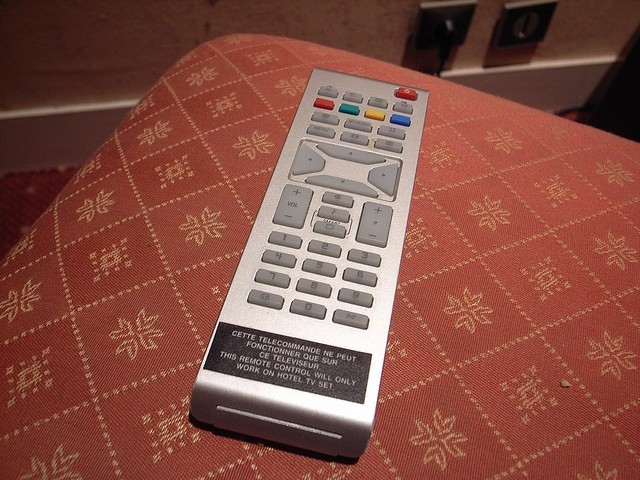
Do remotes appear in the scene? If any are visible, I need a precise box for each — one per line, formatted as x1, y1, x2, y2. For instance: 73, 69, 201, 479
189, 67, 429, 455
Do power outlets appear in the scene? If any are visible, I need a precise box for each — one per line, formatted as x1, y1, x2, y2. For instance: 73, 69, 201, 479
494, 0, 558, 52
412, 2, 478, 51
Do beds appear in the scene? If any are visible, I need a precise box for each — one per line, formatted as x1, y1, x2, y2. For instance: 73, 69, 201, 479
0, 32, 640, 476
